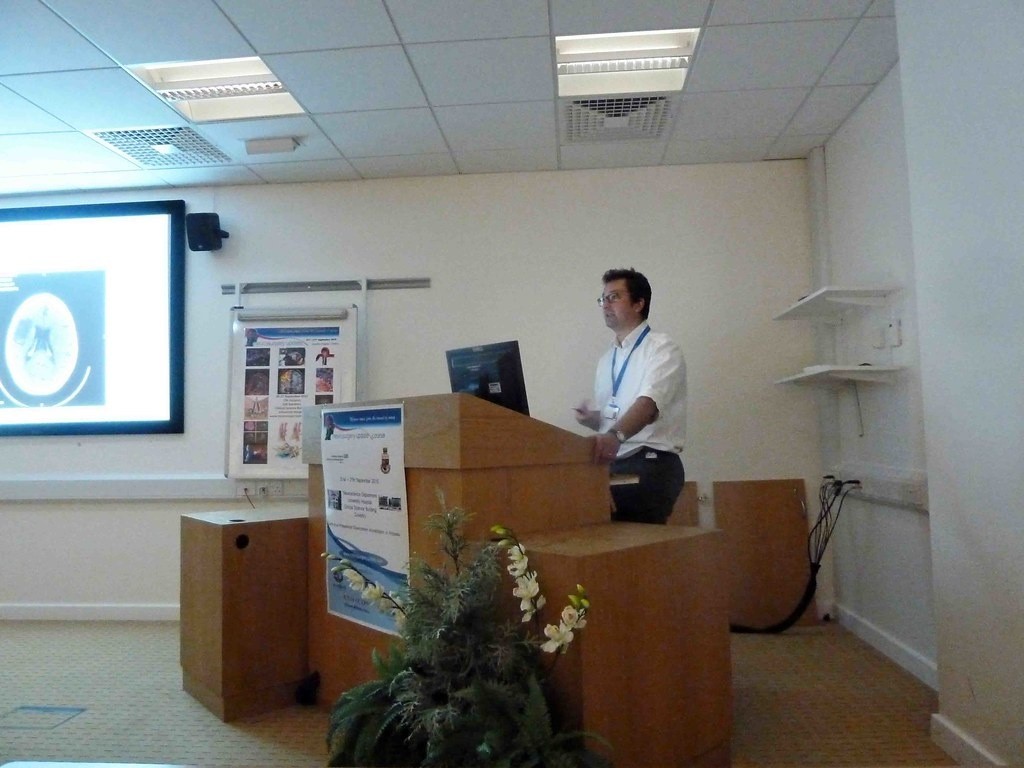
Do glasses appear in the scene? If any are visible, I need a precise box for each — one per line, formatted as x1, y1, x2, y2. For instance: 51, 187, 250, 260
597, 292, 630, 307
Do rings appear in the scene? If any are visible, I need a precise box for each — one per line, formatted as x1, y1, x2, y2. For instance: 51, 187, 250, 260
608, 453, 613, 455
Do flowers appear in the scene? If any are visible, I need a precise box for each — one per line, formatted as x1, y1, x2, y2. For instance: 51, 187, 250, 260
323, 487, 616, 768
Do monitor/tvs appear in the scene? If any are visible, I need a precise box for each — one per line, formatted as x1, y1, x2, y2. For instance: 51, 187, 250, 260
444, 340, 531, 417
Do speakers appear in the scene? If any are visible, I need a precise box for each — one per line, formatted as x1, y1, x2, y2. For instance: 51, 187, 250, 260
186, 212, 223, 252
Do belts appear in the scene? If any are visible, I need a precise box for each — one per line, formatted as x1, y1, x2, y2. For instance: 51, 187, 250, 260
635, 446, 670, 457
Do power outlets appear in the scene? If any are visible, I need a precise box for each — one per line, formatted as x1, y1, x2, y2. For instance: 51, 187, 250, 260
826, 472, 866, 493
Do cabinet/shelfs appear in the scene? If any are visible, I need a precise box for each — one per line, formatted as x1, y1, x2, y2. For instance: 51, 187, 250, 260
774, 286, 894, 388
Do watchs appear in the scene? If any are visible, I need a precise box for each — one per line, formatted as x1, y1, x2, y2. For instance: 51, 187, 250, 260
609, 428, 626, 444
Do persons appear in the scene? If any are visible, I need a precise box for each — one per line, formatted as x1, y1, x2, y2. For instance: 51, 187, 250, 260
572, 267, 686, 527
